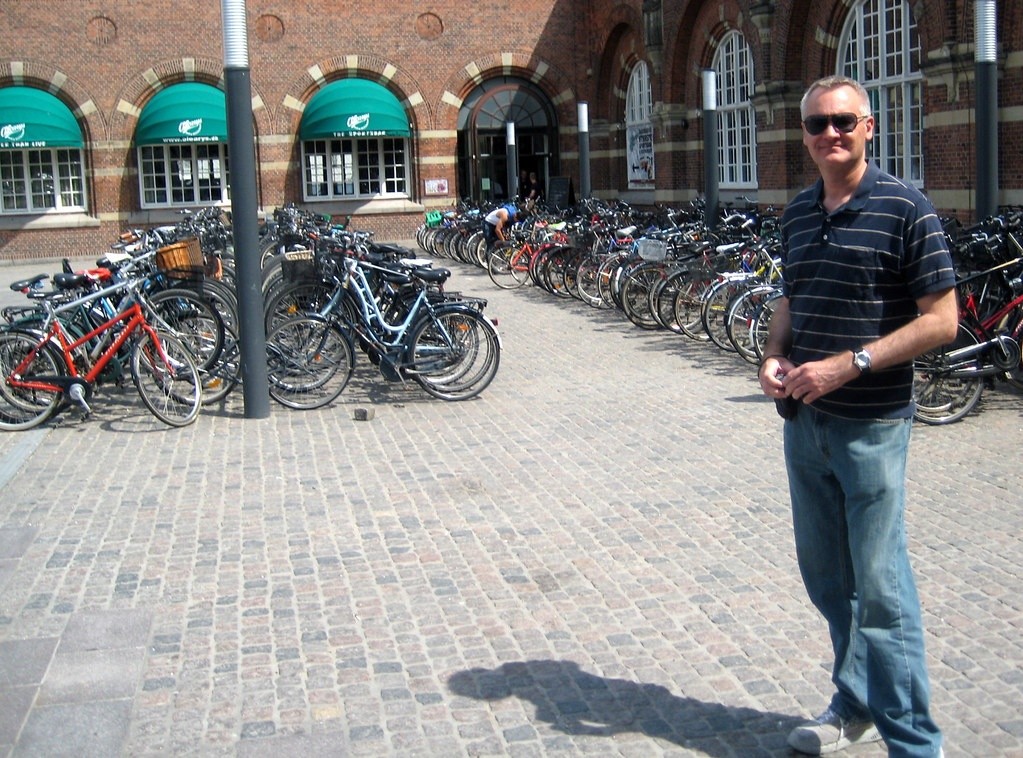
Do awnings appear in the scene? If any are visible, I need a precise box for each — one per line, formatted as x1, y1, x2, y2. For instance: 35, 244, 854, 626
134, 81, 229, 147
0, 86, 85, 149
298, 78, 411, 140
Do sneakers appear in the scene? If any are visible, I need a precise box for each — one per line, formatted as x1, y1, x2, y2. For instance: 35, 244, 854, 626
787, 707, 883, 754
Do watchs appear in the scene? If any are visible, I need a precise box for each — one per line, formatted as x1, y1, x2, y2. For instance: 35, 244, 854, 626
758, 75, 958, 758
847, 344, 873, 377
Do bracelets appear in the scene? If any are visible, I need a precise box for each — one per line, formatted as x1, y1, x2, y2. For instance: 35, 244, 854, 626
758, 353, 791, 378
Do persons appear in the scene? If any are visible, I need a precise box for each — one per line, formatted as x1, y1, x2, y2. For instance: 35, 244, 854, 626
483, 201, 527, 270
520, 170, 545, 203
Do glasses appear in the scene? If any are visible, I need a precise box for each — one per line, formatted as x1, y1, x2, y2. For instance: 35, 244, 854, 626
801, 112, 867, 136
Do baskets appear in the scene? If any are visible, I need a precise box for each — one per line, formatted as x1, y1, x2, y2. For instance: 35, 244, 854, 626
684, 252, 730, 283
280, 260, 313, 284
156, 238, 206, 280
638, 239, 668, 262
168, 263, 206, 308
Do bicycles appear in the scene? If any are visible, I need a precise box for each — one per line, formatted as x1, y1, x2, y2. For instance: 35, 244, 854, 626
416, 188, 785, 362
912, 203, 1023, 424
0, 204, 240, 431
259, 203, 503, 412
